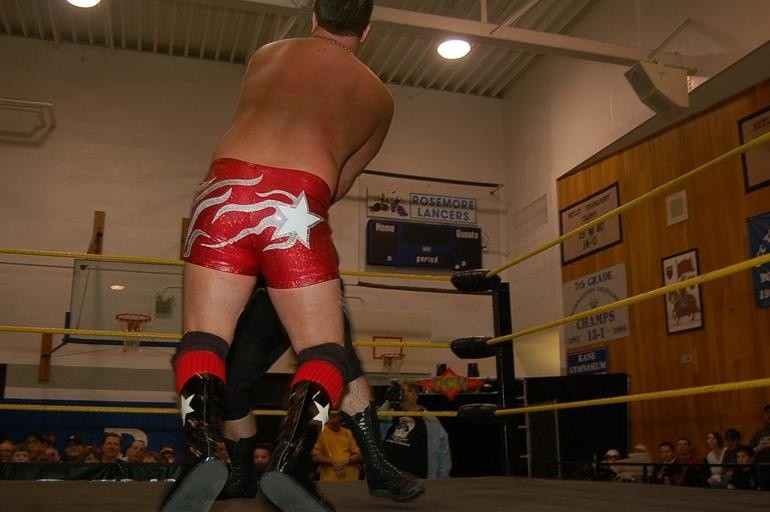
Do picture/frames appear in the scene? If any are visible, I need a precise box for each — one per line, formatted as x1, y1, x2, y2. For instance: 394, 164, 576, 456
736, 105, 770, 194
660, 248, 705, 335
559, 180, 623, 266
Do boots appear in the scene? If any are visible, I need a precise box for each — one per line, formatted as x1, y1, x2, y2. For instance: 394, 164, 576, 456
338, 398, 426, 501
259, 379, 336, 512
215, 430, 258, 501
157, 373, 233, 512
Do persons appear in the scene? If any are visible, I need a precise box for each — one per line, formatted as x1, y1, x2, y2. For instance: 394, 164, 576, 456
2, 433, 274, 463
310, 408, 362, 481
158, 0, 396, 512
377, 381, 452, 480
214, 269, 427, 501
647, 404, 770, 489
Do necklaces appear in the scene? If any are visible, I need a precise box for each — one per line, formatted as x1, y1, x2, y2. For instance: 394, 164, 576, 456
322, 37, 354, 54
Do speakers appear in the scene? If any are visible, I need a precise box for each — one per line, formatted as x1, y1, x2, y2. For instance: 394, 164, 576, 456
624, 62, 689, 118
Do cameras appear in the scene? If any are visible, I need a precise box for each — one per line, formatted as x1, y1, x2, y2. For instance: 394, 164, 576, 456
385, 380, 400, 409
